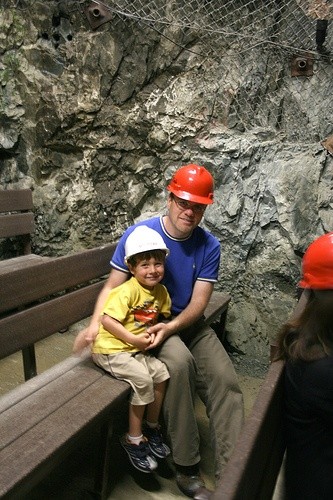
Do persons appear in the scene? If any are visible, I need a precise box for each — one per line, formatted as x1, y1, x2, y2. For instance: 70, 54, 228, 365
276, 231, 333, 500
90, 225, 172, 471
73, 164, 244, 497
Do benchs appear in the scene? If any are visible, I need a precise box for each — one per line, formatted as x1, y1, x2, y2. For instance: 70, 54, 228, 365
0, 189, 51, 277
1, 244, 231, 500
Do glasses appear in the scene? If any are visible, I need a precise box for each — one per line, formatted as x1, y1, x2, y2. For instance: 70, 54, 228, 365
173, 197, 206, 212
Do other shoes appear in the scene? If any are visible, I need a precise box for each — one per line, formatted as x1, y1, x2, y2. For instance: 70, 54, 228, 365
175, 461, 205, 497
143, 422, 171, 459
120, 433, 158, 473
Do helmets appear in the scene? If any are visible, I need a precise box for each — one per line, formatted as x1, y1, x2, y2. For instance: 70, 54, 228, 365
297, 231, 333, 290
123, 224, 170, 261
166, 165, 215, 206
